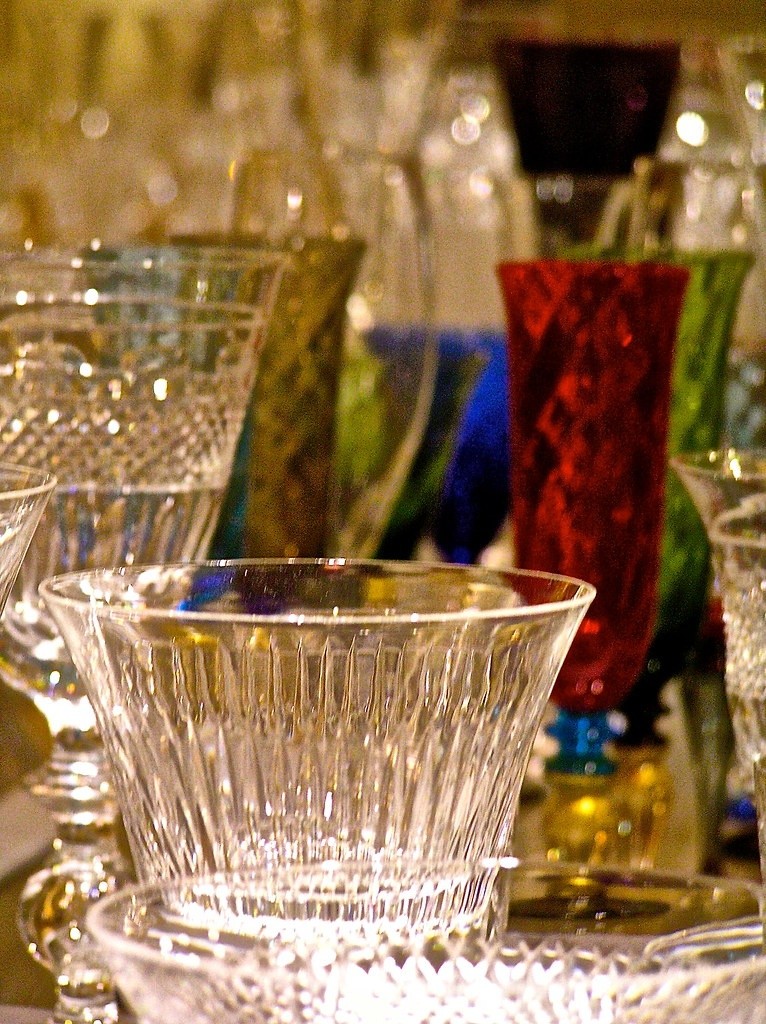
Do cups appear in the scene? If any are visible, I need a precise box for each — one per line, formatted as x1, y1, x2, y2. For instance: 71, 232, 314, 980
1, 0, 765, 1024
